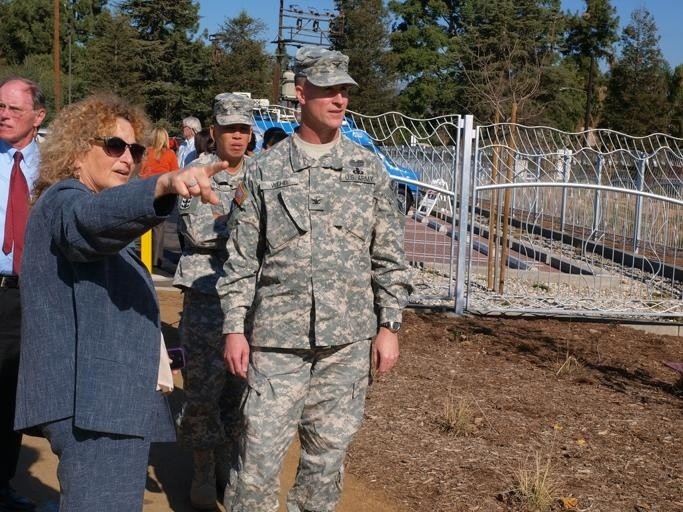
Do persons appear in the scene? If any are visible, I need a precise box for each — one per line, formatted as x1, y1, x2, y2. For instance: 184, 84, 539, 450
129, 117, 301, 281
172, 91, 258, 510
0, 77, 40, 512
213, 46, 413, 511
12, 92, 229, 512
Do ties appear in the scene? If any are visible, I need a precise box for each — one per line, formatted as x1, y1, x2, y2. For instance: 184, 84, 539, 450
2, 151, 32, 274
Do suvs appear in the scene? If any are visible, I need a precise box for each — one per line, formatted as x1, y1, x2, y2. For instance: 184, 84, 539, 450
241, 115, 426, 215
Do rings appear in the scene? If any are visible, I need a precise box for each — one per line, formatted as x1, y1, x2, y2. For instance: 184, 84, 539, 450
187, 180, 197, 188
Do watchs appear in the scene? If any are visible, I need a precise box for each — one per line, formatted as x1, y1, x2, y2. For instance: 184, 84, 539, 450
378, 322, 401, 333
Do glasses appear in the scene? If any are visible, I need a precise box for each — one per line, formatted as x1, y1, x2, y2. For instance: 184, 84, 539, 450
89, 136, 145, 163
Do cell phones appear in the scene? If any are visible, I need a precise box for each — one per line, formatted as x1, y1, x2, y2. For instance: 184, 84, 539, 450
167, 348, 186, 370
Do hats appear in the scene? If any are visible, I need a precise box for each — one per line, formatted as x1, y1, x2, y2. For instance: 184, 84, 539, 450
294, 46, 359, 87
213, 92, 255, 126
